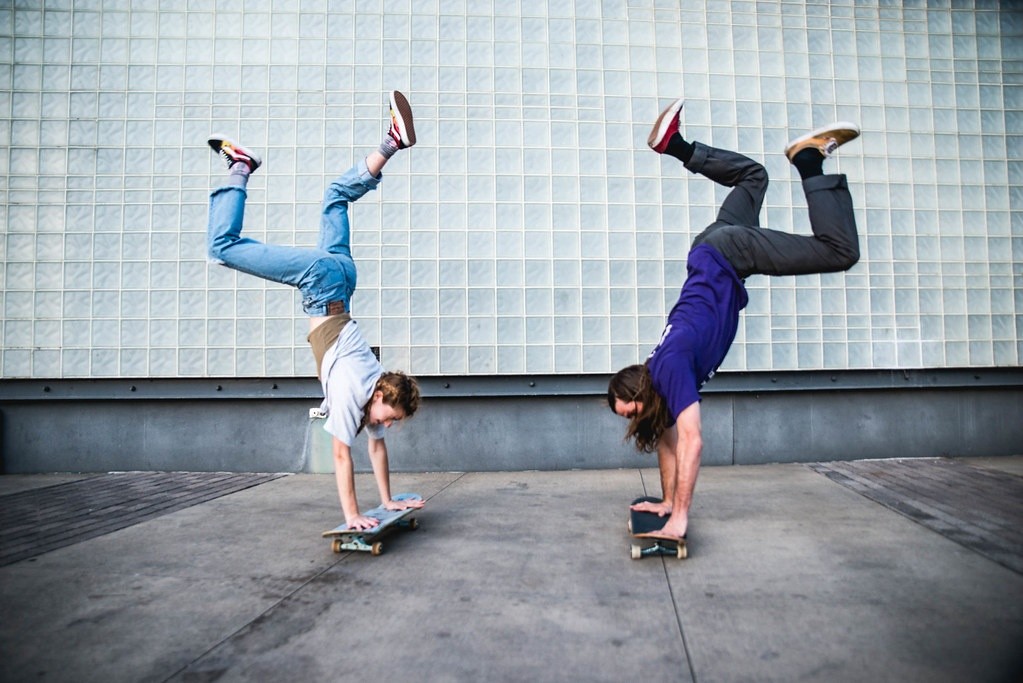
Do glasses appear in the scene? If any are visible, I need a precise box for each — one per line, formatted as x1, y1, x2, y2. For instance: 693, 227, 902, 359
626, 399, 638, 420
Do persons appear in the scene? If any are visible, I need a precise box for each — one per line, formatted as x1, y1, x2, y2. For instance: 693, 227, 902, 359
605, 99, 861, 538
205, 90, 426, 531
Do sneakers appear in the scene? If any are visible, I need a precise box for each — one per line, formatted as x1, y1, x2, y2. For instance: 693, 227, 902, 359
647, 98, 685, 154
386, 90, 416, 149
784, 121, 861, 165
207, 133, 262, 173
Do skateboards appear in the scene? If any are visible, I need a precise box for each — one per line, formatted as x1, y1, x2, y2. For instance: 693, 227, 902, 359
320, 492, 423, 556
627, 496, 689, 560
